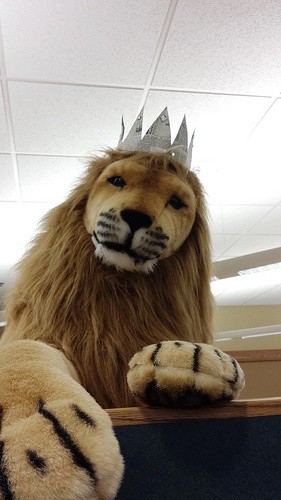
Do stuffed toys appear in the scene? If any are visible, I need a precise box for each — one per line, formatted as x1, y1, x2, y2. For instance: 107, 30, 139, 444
1, 107, 245, 500
